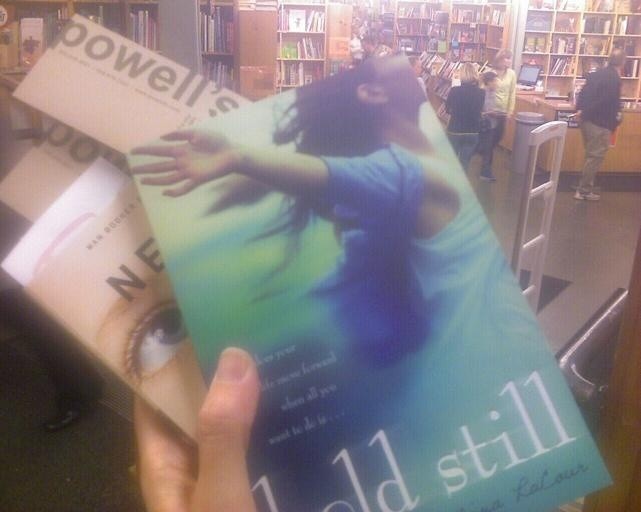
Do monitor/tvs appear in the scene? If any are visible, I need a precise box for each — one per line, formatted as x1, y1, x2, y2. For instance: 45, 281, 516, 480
517, 63, 543, 85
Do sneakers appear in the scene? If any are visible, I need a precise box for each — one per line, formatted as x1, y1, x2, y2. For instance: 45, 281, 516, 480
479, 170, 498, 182
574, 190, 600, 200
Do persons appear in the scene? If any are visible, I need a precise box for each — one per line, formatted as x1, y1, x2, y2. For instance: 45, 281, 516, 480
569, 47, 630, 201
474, 71, 507, 184
494, 49, 520, 147
25, 184, 208, 440
125, 339, 267, 512
442, 59, 483, 173
127, 49, 512, 512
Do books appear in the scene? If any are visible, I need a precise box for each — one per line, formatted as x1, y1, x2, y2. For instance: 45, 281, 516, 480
0, 153, 211, 452
129, 5, 161, 54
0, 121, 129, 222
197, 6, 238, 91
12, 13, 256, 158
125, 50, 616, 512
18, 0, 106, 73
239, 0, 278, 11
277, 8, 327, 93
330, 1, 641, 124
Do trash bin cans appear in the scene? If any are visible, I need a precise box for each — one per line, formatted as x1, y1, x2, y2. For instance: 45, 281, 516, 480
512, 112, 545, 173
